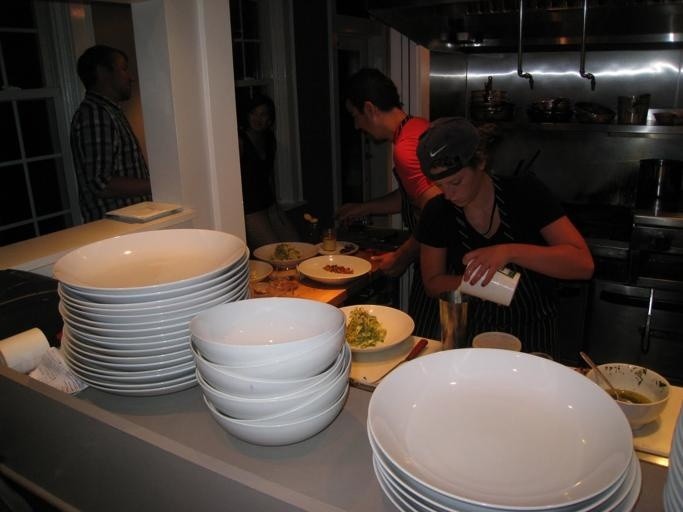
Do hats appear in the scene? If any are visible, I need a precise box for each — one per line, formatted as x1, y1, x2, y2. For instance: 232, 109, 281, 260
416, 115, 496, 181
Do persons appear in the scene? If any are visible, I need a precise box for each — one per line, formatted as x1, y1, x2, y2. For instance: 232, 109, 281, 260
71, 44, 151, 223
238, 96, 278, 246
418, 116, 595, 368
333, 66, 447, 342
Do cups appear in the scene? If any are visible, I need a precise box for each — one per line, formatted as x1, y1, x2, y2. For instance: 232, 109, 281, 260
274, 262, 300, 290
472, 331, 522, 351
438, 291, 469, 352
267, 279, 293, 298
460, 258, 521, 308
321, 227, 337, 251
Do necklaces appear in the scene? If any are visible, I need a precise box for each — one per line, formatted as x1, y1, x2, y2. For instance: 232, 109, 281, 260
481, 176, 497, 236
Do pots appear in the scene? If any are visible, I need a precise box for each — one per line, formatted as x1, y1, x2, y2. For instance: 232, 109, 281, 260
468, 75, 514, 124
528, 96, 570, 120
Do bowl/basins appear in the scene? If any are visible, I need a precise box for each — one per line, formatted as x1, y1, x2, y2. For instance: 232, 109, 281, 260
652, 113, 673, 125
574, 102, 616, 123
189, 298, 351, 445
615, 93, 650, 125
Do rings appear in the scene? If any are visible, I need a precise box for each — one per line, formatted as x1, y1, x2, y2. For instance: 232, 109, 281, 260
465, 269, 473, 276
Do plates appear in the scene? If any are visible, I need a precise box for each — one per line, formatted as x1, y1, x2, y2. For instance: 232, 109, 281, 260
365, 346, 682, 511
314, 241, 359, 255
297, 255, 372, 285
248, 260, 273, 283
253, 242, 318, 269
53, 227, 252, 399
338, 303, 416, 353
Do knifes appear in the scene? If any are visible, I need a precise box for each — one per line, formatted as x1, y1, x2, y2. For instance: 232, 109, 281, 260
371, 338, 429, 384
